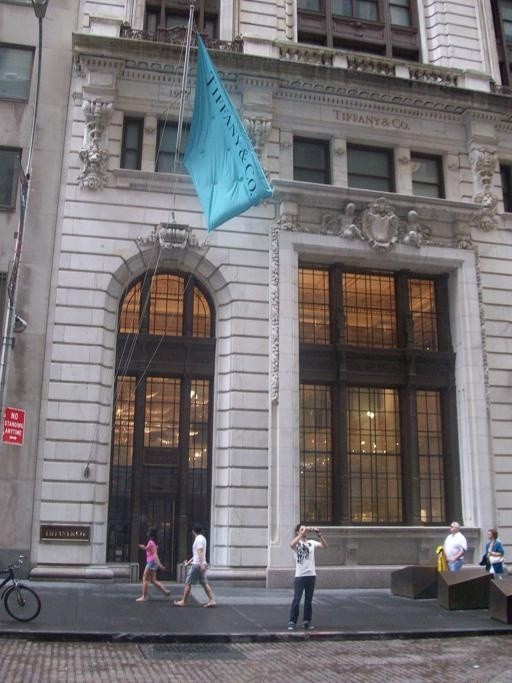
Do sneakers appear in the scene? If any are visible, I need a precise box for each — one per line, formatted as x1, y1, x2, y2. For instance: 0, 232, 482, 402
202, 599, 218, 608
286, 621, 297, 631
135, 594, 150, 602
173, 599, 189, 607
161, 589, 172, 599
301, 620, 316, 630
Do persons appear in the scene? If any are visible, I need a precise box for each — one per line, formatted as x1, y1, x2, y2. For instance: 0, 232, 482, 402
175, 524, 217, 608
288, 524, 328, 629
480, 528, 504, 576
443, 521, 467, 572
135, 528, 170, 602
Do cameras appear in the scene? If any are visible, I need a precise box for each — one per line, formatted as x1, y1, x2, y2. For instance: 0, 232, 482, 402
307, 528, 316, 532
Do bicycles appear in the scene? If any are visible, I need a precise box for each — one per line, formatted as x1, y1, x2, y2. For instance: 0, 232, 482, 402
1, 551, 42, 623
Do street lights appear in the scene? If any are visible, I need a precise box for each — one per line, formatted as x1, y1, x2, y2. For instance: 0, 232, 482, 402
1, 0, 50, 436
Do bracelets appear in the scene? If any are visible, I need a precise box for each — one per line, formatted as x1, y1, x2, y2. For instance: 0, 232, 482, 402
318, 534, 323, 537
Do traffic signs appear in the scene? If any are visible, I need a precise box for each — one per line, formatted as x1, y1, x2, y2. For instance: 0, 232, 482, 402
3, 406, 27, 445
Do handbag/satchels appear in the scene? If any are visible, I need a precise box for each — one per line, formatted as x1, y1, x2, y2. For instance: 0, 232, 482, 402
487, 540, 505, 565
